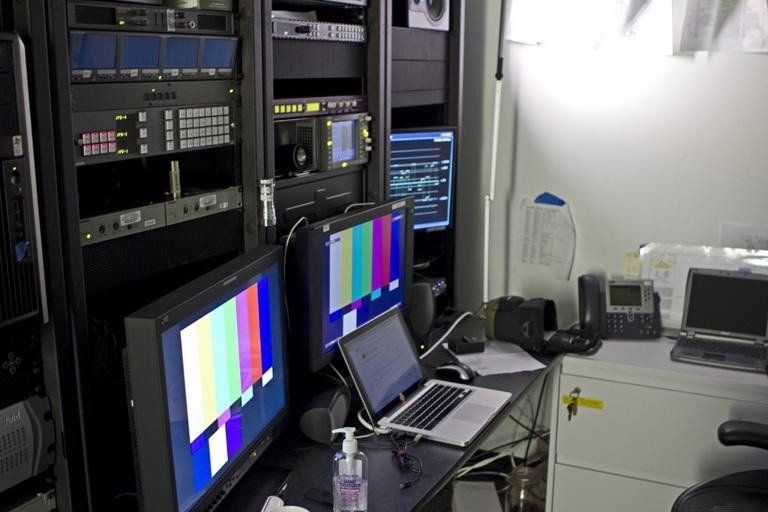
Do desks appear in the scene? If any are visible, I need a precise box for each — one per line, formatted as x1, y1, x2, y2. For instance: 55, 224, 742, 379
288, 353, 563, 507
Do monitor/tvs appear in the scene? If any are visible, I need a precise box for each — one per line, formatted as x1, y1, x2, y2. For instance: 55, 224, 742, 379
390, 125, 458, 230
121, 245, 289, 512
305, 195, 415, 373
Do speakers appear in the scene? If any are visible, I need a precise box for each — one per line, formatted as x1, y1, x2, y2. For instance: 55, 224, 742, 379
404, 283, 435, 336
299, 387, 350, 445
403, 2, 451, 32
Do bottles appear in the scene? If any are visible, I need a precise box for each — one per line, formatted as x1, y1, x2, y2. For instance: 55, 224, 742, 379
502, 465, 546, 511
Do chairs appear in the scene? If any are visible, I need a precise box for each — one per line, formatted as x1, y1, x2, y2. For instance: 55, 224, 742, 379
660, 416, 765, 512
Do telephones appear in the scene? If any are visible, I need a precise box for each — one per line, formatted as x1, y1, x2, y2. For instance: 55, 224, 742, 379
578, 275, 661, 339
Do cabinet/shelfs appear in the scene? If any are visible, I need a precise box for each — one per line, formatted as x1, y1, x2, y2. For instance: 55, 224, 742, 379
544, 328, 766, 510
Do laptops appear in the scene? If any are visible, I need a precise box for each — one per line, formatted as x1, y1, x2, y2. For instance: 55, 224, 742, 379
671, 267, 768, 375
337, 305, 512, 447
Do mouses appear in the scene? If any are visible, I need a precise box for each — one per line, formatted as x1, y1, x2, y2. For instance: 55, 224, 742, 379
432, 360, 473, 383
